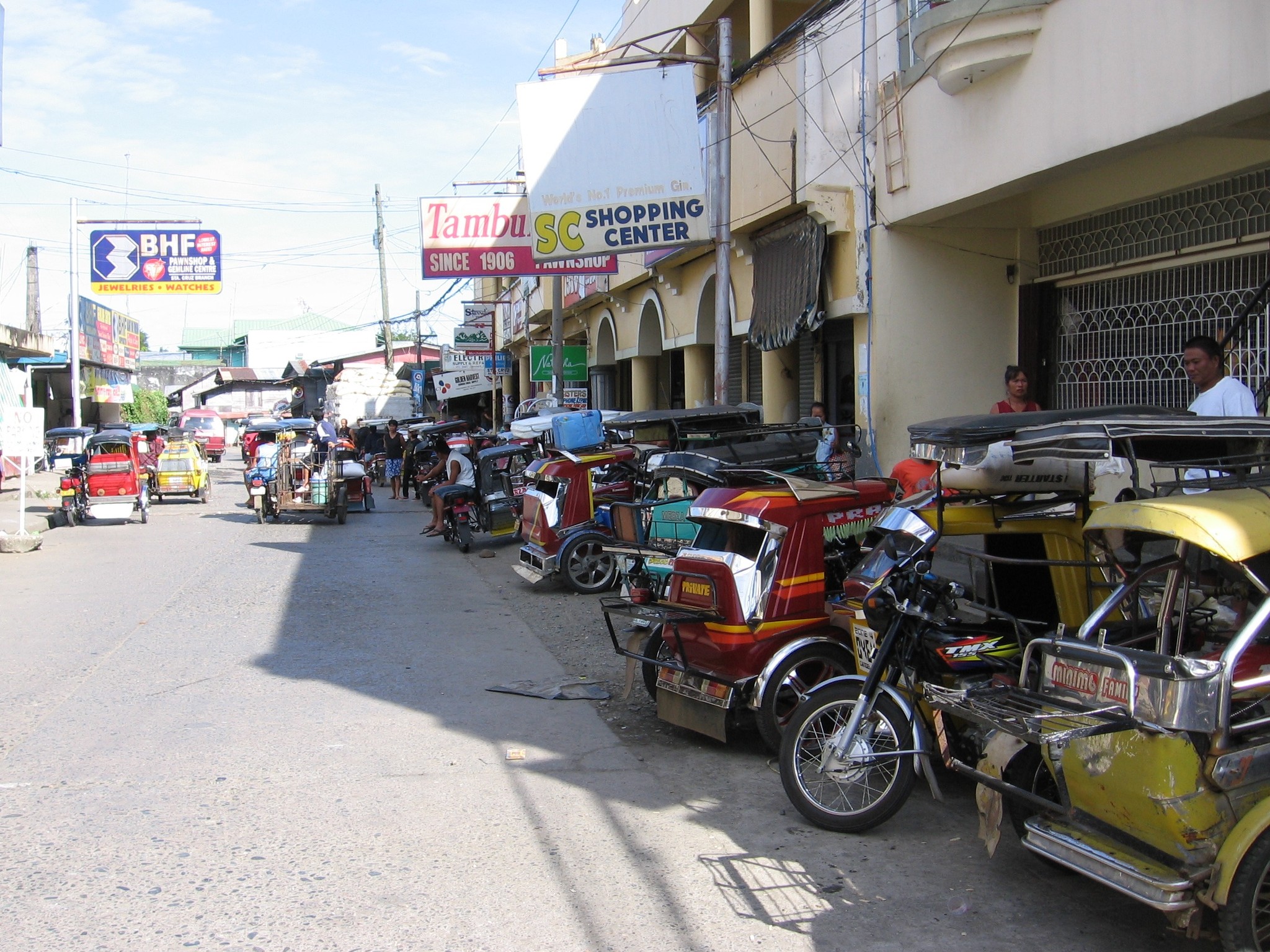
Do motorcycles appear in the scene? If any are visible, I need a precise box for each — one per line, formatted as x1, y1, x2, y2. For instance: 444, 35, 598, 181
337, 416, 550, 553
44, 423, 211, 524
57, 466, 90, 526
511, 403, 1269, 952
244, 457, 273, 524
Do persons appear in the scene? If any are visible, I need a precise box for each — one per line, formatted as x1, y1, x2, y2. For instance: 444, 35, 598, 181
1181, 334, 1258, 645
810, 401, 839, 481
244, 406, 422, 506
989, 365, 1041, 414
156, 430, 165, 449
452, 407, 669, 473
416, 439, 476, 537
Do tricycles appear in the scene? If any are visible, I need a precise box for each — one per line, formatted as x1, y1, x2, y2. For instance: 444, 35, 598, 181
268, 419, 348, 524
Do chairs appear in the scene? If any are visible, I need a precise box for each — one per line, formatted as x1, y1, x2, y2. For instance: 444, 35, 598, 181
188, 417, 213, 428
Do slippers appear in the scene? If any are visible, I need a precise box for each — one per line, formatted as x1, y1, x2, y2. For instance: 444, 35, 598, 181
296, 486, 310, 492
419, 526, 435, 534
426, 528, 447, 537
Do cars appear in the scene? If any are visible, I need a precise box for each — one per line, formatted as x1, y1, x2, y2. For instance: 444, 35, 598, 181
239, 425, 280, 463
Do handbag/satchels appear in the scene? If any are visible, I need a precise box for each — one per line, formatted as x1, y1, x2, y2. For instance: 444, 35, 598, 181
828, 447, 855, 480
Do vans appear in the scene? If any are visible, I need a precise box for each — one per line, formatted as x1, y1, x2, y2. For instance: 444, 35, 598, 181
172, 409, 226, 463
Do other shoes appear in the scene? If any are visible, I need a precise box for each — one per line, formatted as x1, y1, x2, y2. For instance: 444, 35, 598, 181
412, 498, 420, 500
395, 497, 401, 500
389, 497, 395, 499
403, 497, 408, 499
